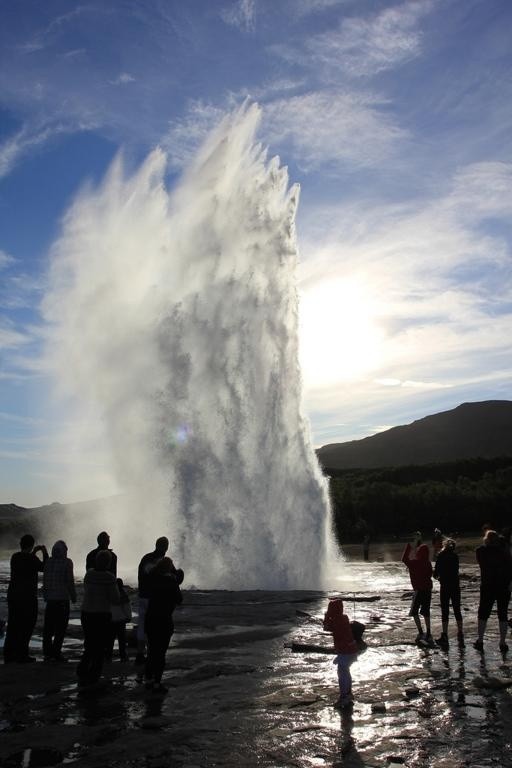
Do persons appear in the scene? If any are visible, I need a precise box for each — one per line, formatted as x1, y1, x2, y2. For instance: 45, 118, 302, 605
4, 527, 46, 661
322, 598, 357, 709
399, 524, 511, 655
356, 518, 374, 561
39, 540, 77, 665
81, 530, 185, 697
